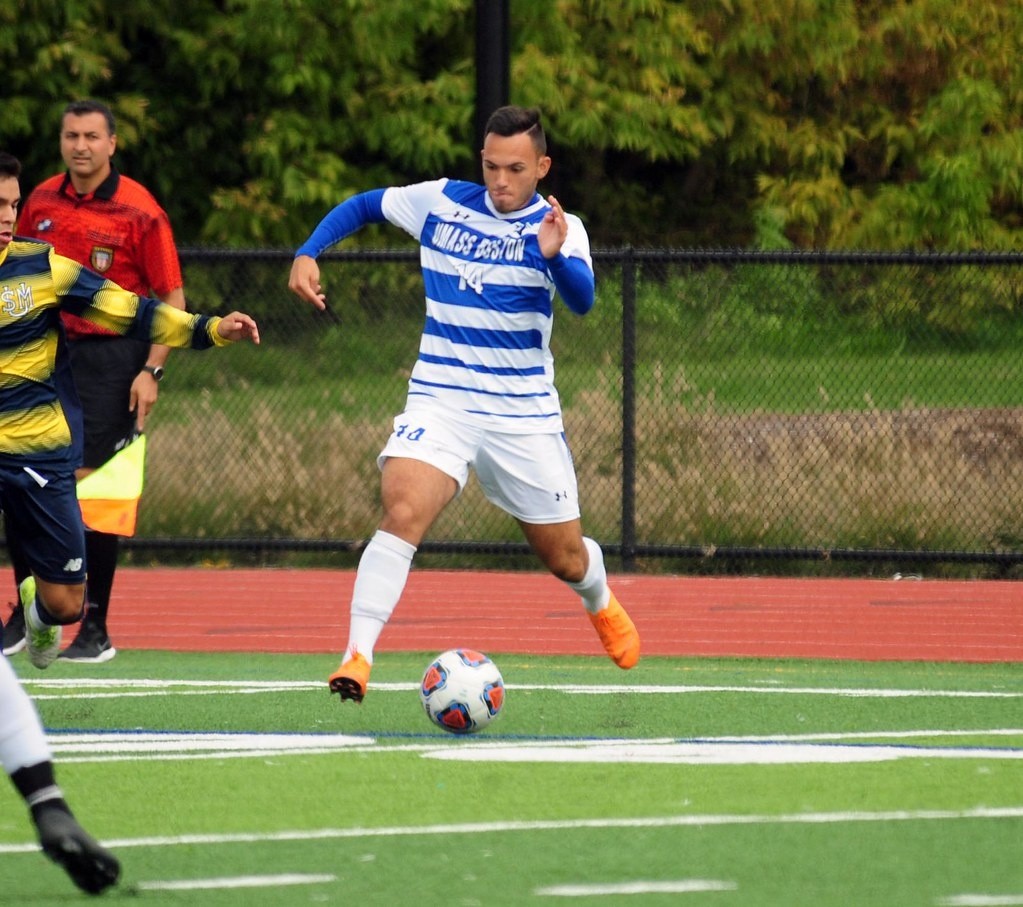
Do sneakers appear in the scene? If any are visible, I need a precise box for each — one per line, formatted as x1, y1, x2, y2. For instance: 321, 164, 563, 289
586, 591, 640, 670
37, 807, 120, 897
57, 618, 116, 663
329, 643, 372, 704
3, 601, 25, 655
19, 576, 60, 669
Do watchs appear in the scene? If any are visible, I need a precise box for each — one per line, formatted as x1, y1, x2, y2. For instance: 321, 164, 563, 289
141, 364, 165, 379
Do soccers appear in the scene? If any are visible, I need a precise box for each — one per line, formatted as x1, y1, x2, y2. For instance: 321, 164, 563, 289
421, 649, 505, 734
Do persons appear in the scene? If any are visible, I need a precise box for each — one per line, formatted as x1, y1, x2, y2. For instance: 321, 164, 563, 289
16, 99, 187, 663
0, 153, 260, 675
0, 649, 120, 899
289, 103, 642, 709
